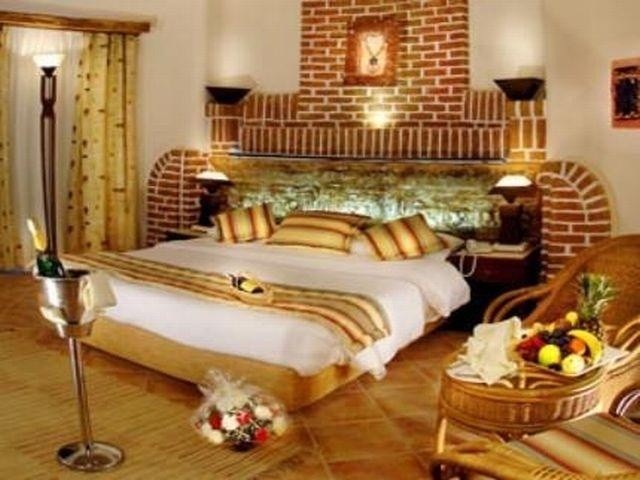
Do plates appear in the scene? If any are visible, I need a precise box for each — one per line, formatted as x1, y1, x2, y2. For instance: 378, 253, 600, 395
515, 342, 629, 377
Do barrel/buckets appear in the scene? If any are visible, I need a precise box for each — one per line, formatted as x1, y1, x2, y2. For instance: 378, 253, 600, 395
34, 270, 95, 338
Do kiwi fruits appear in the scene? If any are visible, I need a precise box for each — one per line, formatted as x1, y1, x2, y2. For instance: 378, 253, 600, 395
554, 318, 572, 332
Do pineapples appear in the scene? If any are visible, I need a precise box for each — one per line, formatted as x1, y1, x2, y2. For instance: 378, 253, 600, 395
576, 271, 620, 350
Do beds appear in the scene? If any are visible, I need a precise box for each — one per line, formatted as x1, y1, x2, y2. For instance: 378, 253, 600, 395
50, 227, 463, 415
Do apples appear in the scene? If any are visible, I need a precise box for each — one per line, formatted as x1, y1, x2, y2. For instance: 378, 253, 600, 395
538, 344, 561, 366
567, 312, 579, 326
561, 354, 586, 374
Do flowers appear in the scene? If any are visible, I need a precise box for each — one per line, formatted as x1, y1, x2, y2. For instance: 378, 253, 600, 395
191, 369, 290, 453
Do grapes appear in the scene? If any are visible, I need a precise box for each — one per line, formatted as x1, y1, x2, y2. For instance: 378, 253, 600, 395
521, 329, 570, 371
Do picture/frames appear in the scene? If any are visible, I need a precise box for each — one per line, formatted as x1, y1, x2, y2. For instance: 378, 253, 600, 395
342, 13, 400, 87
607, 55, 640, 131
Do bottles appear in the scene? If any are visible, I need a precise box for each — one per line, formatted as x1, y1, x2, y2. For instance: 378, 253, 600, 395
26, 214, 69, 280
227, 271, 266, 294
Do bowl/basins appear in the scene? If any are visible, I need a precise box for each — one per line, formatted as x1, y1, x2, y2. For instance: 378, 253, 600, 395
207, 84, 252, 105
492, 77, 547, 101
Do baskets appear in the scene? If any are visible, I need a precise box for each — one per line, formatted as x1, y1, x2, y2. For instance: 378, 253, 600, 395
228, 269, 275, 306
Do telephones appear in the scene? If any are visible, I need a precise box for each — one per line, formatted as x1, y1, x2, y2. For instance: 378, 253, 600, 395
466, 239, 493, 254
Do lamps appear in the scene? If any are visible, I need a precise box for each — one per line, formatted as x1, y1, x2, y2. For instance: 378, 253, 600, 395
190, 169, 234, 227
488, 175, 537, 242
31, 50, 66, 259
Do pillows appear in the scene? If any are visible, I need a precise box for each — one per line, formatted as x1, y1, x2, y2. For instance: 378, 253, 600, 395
210, 202, 448, 261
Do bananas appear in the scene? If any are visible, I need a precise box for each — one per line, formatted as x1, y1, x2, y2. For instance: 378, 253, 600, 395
567, 329, 603, 367
